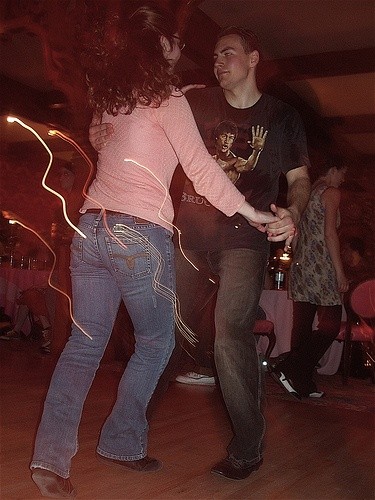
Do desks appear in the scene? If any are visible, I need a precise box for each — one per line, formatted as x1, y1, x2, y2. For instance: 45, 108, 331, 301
0, 261, 348, 376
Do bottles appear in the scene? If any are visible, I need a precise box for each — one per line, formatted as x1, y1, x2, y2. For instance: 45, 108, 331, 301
264, 256, 286, 290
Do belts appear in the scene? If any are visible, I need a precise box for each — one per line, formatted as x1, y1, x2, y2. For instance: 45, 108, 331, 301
83, 208, 115, 213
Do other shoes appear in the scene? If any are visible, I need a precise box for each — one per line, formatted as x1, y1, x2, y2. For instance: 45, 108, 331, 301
0, 329, 21, 339
41, 338, 51, 348
42, 346, 51, 353
271, 368, 302, 401
309, 390, 326, 398
175, 372, 216, 386
96, 455, 162, 472
31, 468, 77, 498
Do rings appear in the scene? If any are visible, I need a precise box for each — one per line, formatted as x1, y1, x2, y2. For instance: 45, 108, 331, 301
343, 287, 346, 290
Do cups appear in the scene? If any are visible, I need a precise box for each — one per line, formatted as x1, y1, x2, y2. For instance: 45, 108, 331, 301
0, 255, 47, 272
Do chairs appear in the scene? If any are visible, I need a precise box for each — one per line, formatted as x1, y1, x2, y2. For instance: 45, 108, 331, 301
191, 265, 277, 375
335, 276, 375, 387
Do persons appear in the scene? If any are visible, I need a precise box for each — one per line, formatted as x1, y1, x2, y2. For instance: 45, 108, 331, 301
342, 237, 373, 379
272, 154, 348, 400
211, 121, 268, 184
0, 166, 75, 353
177, 352, 214, 385
28, 5, 279, 497
88, 24, 312, 483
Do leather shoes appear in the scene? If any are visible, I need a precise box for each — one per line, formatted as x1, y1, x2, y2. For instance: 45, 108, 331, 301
209, 454, 263, 481
